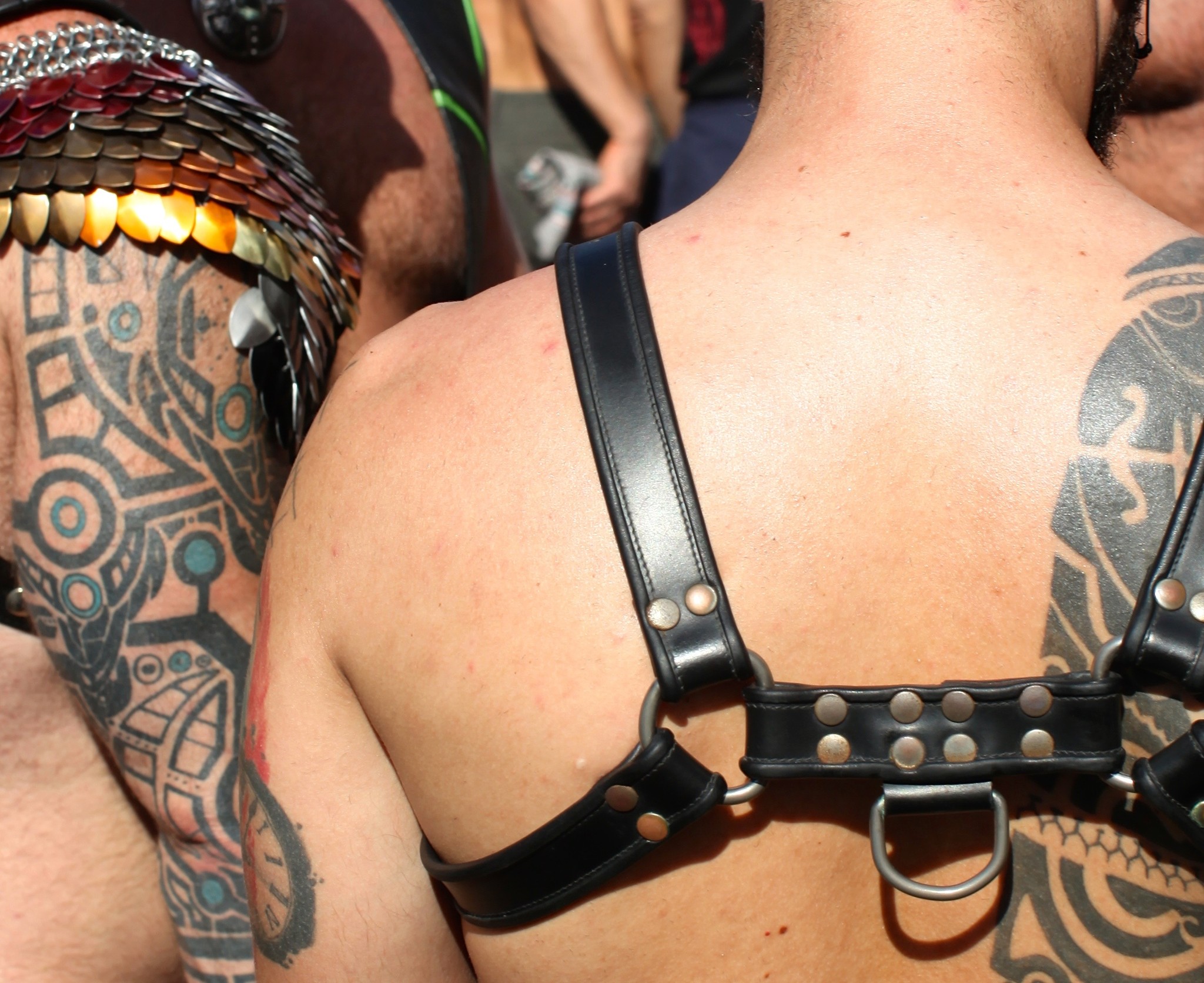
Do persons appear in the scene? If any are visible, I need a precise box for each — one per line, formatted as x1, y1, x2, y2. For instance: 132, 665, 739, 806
4, 1, 1202, 982
